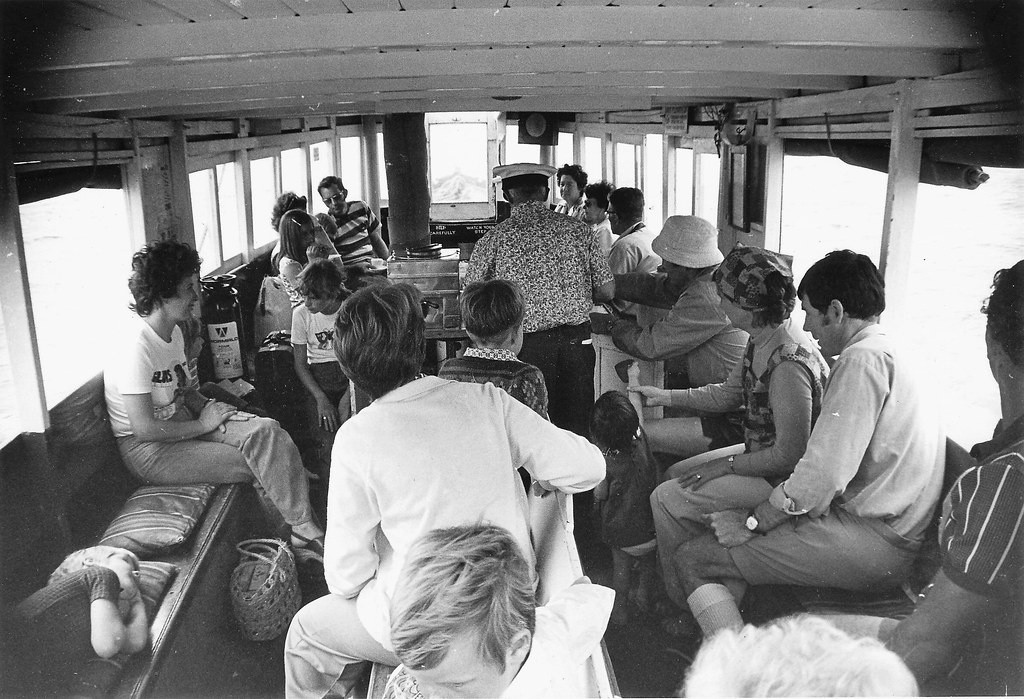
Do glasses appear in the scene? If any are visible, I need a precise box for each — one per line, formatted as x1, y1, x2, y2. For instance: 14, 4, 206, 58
323, 188, 344, 206
585, 200, 605, 210
604, 210, 616, 219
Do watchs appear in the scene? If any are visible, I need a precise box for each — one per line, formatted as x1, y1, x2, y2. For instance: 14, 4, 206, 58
746, 508, 767, 537
727, 455, 737, 476
204, 398, 213, 408
607, 317, 620, 336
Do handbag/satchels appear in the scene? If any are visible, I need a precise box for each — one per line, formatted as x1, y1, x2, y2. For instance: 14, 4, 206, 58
230, 539, 302, 642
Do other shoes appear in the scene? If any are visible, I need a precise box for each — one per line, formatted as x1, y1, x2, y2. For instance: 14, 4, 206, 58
666, 612, 702, 643
647, 597, 679, 618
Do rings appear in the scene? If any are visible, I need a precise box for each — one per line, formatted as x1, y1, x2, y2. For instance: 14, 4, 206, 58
323, 416, 327, 420
696, 473, 702, 480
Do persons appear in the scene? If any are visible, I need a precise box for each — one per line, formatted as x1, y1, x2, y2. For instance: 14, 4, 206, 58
176, 312, 322, 485
589, 214, 750, 418
679, 612, 921, 697
463, 163, 615, 438
812, 259, 1023, 697
316, 213, 352, 301
382, 523, 615, 698
674, 249, 947, 642
554, 164, 588, 221
318, 176, 390, 289
1, 546, 150, 698
277, 208, 326, 309
270, 192, 344, 273
102, 236, 326, 565
438, 280, 552, 424
583, 181, 620, 261
290, 260, 358, 492
604, 187, 668, 329
589, 361, 662, 627
285, 283, 607, 699
626, 242, 831, 610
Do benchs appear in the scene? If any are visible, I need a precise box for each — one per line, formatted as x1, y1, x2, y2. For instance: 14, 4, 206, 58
0, 264, 296, 699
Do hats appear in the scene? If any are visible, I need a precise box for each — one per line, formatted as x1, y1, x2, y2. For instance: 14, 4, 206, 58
652, 215, 726, 268
492, 163, 557, 180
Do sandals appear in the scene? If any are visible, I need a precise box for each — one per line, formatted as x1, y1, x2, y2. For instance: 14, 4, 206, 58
290, 530, 325, 565
